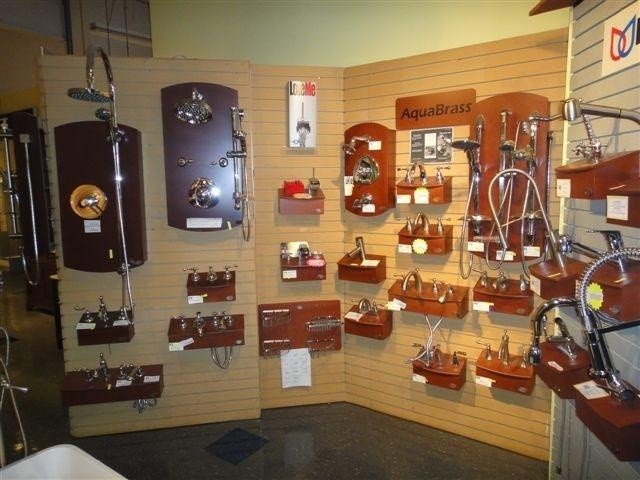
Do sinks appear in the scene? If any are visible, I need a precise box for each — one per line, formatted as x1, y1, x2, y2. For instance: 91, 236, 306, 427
0, 443, 130, 480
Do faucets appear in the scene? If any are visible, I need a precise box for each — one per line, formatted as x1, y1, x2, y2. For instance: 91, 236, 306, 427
429, 348, 443, 366
409, 161, 429, 184
555, 226, 631, 275
297, 243, 310, 266
192, 311, 206, 338
487, 168, 570, 272
358, 297, 372, 312
412, 210, 430, 235
491, 271, 508, 291
496, 330, 510, 364
206, 266, 218, 282
541, 314, 578, 360
402, 269, 424, 296
347, 236, 366, 265
438, 283, 453, 305
